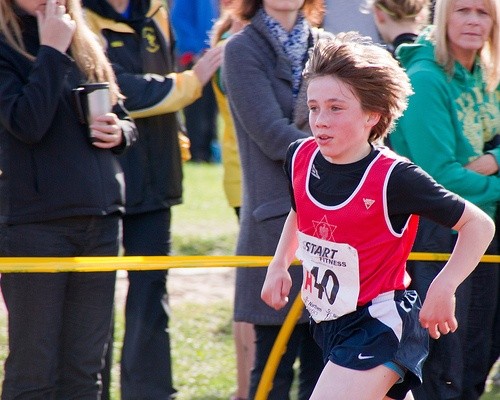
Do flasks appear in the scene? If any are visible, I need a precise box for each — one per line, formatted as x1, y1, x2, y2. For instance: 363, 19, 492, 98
72, 82, 113, 145
